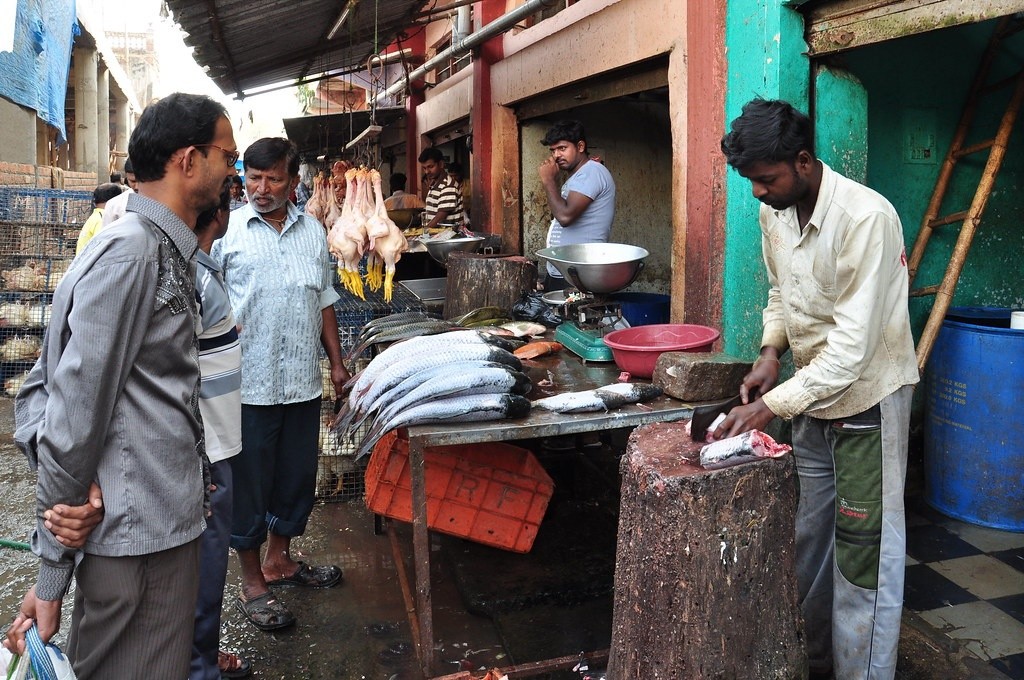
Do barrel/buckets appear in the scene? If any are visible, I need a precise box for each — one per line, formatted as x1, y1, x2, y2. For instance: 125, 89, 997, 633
923, 303, 1024, 534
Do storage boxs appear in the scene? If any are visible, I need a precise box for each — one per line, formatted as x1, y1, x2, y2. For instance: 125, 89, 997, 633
362, 431, 550, 554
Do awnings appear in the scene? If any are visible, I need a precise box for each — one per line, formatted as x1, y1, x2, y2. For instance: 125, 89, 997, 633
282, 107, 408, 149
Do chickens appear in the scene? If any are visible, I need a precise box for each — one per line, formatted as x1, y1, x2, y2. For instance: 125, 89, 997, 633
0, 261, 67, 397
303, 166, 408, 303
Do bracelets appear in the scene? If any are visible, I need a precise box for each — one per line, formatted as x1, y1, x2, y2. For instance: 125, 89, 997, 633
752, 355, 780, 369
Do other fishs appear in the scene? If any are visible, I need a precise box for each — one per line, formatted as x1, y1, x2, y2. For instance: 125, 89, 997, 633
330, 311, 664, 464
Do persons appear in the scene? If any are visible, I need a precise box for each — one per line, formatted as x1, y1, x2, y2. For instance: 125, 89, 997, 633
538, 118, 616, 293
3, 92, 253, 680
211, 137, 351, 632
384, 147, 471, 230
713, 97, 920, 680
74, 156, 310, 255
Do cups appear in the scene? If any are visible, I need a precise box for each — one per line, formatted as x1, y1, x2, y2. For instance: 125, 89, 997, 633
1009, 309, 1024, 330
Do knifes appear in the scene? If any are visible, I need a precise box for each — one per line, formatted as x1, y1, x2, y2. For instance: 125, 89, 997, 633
691, 384, 760, 443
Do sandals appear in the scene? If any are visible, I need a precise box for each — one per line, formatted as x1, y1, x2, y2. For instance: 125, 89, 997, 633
217, 649, 251, 676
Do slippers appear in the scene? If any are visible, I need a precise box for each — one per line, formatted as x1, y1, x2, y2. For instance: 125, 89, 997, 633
266, 560, 343, 588
235, 590, 295, 629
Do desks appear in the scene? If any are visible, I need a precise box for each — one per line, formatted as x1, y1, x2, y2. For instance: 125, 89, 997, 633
373, 326, 732, 680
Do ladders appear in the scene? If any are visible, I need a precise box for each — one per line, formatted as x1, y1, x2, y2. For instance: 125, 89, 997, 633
908, 13, 1024, 391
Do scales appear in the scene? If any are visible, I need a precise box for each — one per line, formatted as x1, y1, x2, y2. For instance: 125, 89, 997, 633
535, 241, 650, 362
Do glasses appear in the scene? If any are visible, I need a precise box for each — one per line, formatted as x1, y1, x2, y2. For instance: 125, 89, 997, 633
178, 144, 240, 167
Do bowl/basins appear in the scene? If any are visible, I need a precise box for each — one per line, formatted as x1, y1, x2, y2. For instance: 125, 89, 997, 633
603, 323, 720, 378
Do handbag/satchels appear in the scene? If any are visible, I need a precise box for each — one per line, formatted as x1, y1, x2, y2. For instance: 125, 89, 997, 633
0, 619, 77, 680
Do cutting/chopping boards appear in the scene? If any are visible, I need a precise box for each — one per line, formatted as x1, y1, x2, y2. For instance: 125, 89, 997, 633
444, 251, 538, 320
